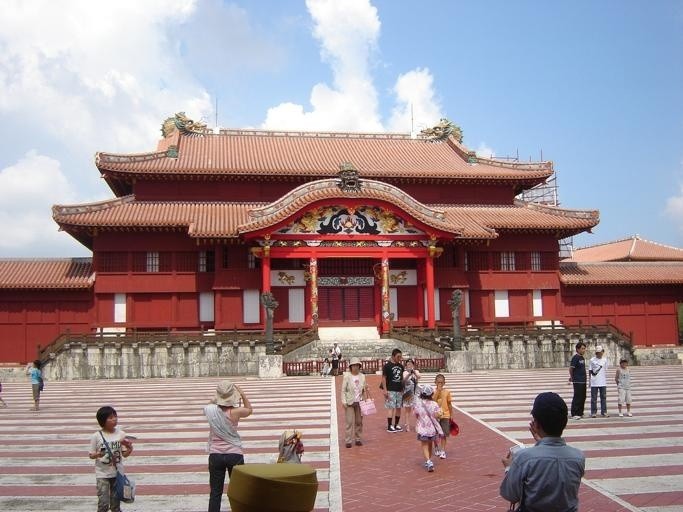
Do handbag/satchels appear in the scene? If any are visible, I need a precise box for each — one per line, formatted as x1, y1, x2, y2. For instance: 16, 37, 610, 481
99, 430, 136, 503
420, 400, 447, 449
36, 370, 44, 390
359, 389, 377, 416
334, 348, 342, 359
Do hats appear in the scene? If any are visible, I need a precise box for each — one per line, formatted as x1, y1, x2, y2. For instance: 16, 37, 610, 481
532, 390, 567, 418
333, 339, 340, 343
595, 345, 603, 354
620, 357, 627, 363
406, 358, 414, 364
421, 384, 434, 396
349, 357, 362, 366
447, 419, 459, 436
217, 380, 239, 407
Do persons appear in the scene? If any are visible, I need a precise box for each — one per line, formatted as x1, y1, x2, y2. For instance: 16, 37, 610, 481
588, 346, 610, 418
321, 357, 329, 377
203, 381, 252, 512
569, 342, 587, 419
26, 360, 42, 410
0, 381, 6, 409
341, 357, 368, 448
500, 392, 585, 512
89, 406, 134, 512
334, 341, 341, 357
615, 357, 633, 417
414, 385, 447, 472
431, 374, 453, 459
380, 348, 403, 433
402, 359, 421, 432
329, 349, 333, 362
332, 353, 339, 376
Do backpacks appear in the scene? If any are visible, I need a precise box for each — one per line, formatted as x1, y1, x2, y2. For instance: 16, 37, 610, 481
39, 377, 44, 391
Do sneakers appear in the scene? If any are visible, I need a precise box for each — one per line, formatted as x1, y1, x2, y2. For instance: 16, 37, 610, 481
355, 441, 363, 446
387, 425, 396, 433
580, 414, 587, 418
395, 425, 403, 432
618, 413, 623, 416
601, 413, 609, 417
434, 449, 440, 456
589, 414, 597, 417
428, 462, 434, 472
626, 412, 632, 417
405, 424, 410, 428
345, 442, 352, 448
425, 461, 428, 467
572, 415, 581, 420
440, 452, 448, 459
406, 427, 410, 432
